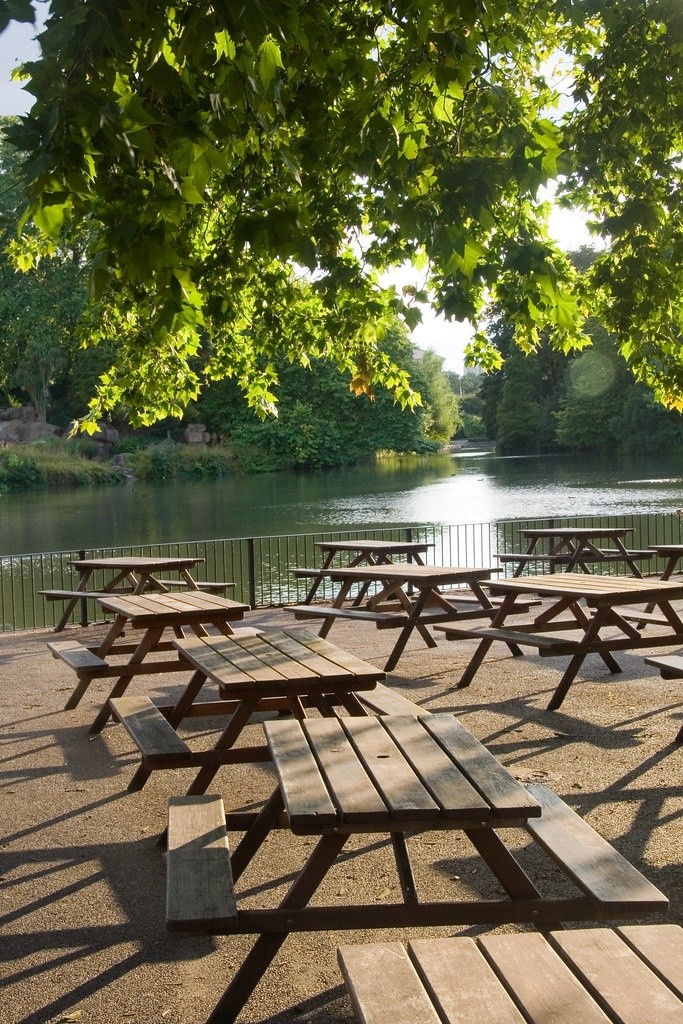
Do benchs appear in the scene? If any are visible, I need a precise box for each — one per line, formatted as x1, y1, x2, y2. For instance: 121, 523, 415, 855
40, 548, 683, 936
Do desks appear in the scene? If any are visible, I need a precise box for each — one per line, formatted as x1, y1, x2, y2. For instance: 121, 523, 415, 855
512, 528, 644, 578
65, 590, 252, 733
317, 562, 524, 672
457, 572, 683, 710
206, 712, 646, 1023
304, 539, 444, 606
54, 557, 207, 634
129, 627, 389, 848
636, 545, 683, 629
338, 923, 683, 1024
